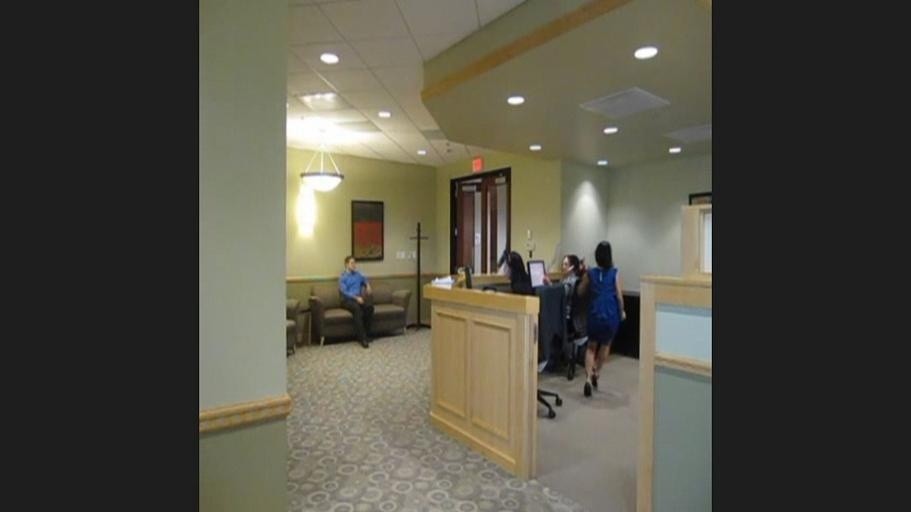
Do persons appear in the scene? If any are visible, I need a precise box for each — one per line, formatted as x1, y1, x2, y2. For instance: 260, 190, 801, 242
339, 256, 374, 347
497, 241, 627, 397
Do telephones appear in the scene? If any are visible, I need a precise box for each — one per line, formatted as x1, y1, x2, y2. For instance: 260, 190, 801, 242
566, 265, 575, 273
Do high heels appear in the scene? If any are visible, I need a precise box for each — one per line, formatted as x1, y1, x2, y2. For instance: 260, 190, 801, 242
583, 375, 597, 397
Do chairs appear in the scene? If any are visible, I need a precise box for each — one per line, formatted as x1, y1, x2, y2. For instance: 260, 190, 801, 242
287, 298, 301, 355
558, 278, 589, 380
532, 285, 564, 419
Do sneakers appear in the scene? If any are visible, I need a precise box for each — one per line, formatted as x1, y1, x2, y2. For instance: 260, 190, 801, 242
362, 333, 370, 348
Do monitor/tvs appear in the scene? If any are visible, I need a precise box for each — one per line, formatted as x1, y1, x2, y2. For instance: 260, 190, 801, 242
526, 260, 546, 288
465, 266, 473, 289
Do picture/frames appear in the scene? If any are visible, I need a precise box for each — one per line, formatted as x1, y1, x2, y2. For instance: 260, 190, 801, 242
351, 199, 384, 261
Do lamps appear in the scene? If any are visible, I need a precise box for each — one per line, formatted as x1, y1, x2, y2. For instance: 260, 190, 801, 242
299, 143, 344, 193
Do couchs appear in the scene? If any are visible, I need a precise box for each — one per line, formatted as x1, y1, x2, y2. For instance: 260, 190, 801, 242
308, 282, 413, 348
